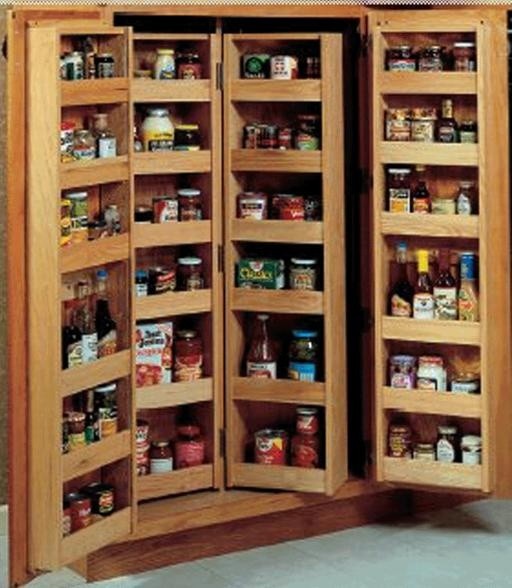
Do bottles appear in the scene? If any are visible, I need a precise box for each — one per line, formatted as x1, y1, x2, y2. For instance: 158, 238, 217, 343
456, 120, 477, 144
433, 425, 460, 463
452, 42, 476, 72
176, 189, 202, 221
64, 192, 87, 244
430, 198, 456, 215
133, 207, 152, 224
148, 440, 173, 474
417, 45, 443, 72
456, 181, 473, 215
153, 48, 176, 81
174, 52, 203, 80
386, 241, 413, 317
457, 251, 479, 321
413, 443, 435, 462
90, 113, 116, 158
71, 278, 99, 365
389, 354, 416, 389
134, 272, 147, 296
387, 169, 410, 213
384, 46, 416, 72
95, 269, 118, 359
174, 403, 206, 468
460, 435, 481, 464
412, 107, 435, 140
388, 424, 411, 457
412, 249, 434, 320
175, 257, 203, 291
172, 124, 200, 151
433, 249, 458, 321
287, 329, 318, 382
173, 330, 203, 382
104, 203, 120, 236
141, 108, 174, 152
289, 257, 316, 290
415, 355, 447, 392
289, 407, 319, 468
246, 315, 278, 379
383, 107, 409, 142
152, 195, 178, 223
435, 99, 460, 144
410, 166, 431, 212
63, 50, 84, 81
62, 308, 82, 371
84, 390, 94, 442
293, 113, 319, 150
94, 383, 118, 440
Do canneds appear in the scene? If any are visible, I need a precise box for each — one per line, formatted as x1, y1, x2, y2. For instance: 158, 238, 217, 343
65, 493, 89, 532
73, 129, 96, 161
253, 429, 287, 464
245, 54, 269, 78
92, 485, 115, 515
448, 373, 478, 392
270, 56, 297, 81
237, 191, 268, 220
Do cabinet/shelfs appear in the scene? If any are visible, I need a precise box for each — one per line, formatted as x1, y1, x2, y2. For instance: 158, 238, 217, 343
0, 0, 511, 588
